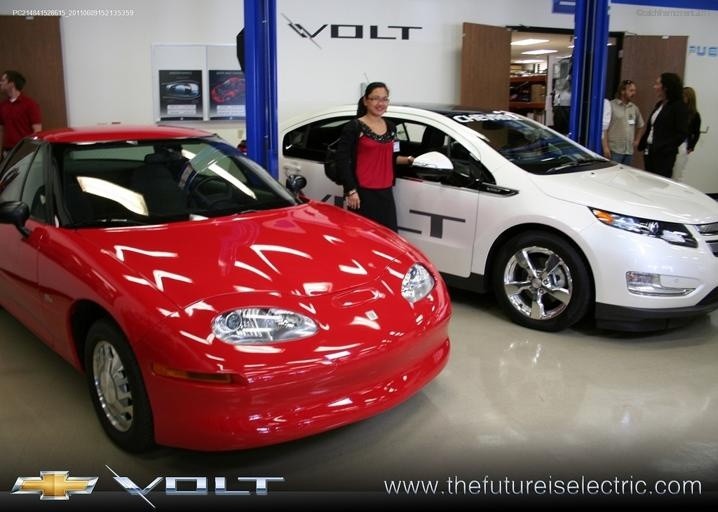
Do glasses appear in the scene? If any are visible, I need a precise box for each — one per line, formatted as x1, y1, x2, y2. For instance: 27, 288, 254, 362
625, 79, 634, 84
365, 97, 390, 104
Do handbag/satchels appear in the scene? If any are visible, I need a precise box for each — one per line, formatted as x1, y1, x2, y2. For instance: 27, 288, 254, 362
325, 119, 361, 185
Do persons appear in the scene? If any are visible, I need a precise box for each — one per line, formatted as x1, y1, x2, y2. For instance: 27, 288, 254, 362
636, 71, 691, 180
338, 81, 418, 239
0, 68, 43, 165
603, 79, 645, 167
671, 86, 702, 181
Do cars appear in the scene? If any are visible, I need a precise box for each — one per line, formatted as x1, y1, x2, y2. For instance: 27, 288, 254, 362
1, 123, 451, 453
211, 78, 245, 104
161, 80, 200, 100
238, 104, 718, 332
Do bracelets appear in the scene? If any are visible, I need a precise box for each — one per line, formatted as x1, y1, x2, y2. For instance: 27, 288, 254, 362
407, 155, 413, 165
345, 189, 357, 197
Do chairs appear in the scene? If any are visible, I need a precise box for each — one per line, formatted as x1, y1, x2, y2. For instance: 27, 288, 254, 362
60, 151, 198, 221
